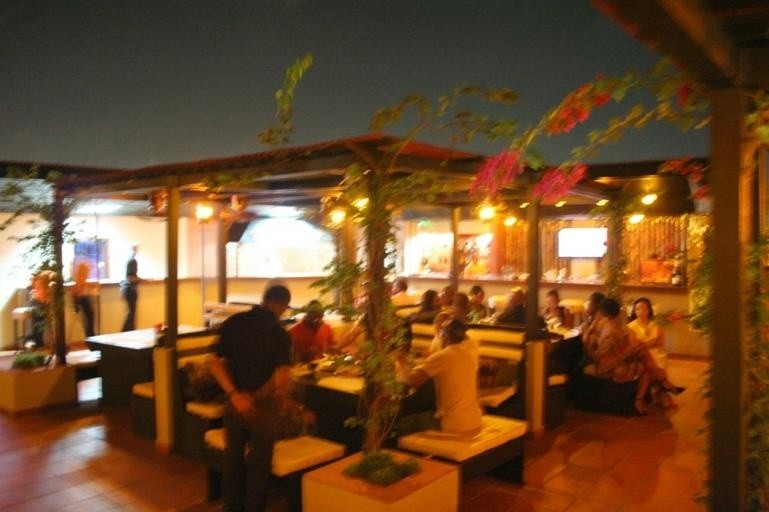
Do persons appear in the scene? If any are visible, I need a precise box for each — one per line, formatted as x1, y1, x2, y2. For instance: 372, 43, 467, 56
208, 284, 334, 509
337, 276, 486, 358
27, 257, 55, 347
405, 306, 482, 432
495, 288, 685, 418
72, 242, 100, 350
118, 244, 147, 331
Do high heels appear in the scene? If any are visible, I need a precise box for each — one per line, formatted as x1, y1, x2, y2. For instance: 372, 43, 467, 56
631, 378, 688, 415
